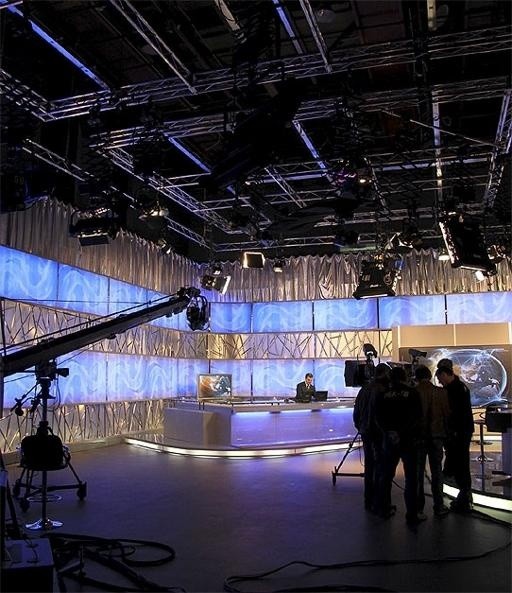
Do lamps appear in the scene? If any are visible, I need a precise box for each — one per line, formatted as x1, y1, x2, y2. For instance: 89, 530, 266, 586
67, 179, 122, 246
350, 257, 396, 301
1, 127, 38, 214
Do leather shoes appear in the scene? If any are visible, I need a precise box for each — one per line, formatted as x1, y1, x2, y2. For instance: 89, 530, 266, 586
386, 505, 396, 520
450, 492, 473, 515
405, 513, 428, 525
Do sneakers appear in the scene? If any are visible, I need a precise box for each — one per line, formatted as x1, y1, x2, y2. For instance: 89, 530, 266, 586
433, 504, 451, 518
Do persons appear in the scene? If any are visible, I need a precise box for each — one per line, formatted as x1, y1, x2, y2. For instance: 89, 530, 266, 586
295, 372, 317, 403
352, 359, 475, 527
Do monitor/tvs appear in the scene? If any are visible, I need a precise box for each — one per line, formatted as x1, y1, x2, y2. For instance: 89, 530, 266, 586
197, 373, 233, 399
315, 391, 328, 400
